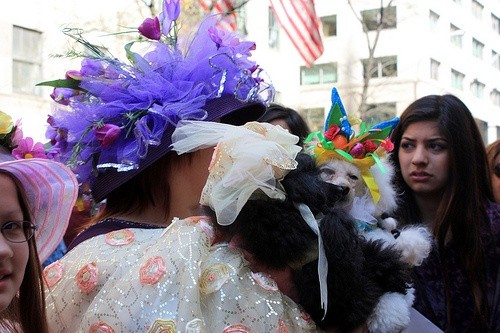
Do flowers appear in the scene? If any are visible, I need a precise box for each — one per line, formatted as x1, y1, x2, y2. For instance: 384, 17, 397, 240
0, 110, 46, 162
32, 0, 278, 192
315, 119, 395, 167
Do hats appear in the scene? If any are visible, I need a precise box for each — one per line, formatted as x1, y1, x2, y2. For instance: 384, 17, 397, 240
35, 0, 276, 203
0, 110, 79, 266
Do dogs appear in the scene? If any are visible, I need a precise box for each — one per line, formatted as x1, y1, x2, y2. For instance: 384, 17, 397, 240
203, 152, 433, 333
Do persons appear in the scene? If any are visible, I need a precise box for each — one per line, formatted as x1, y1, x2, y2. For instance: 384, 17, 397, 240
0, 0, 500, 333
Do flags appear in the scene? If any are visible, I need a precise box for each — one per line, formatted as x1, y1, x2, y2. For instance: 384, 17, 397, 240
269, 0, 324, 68
199, 0, 238, 34
